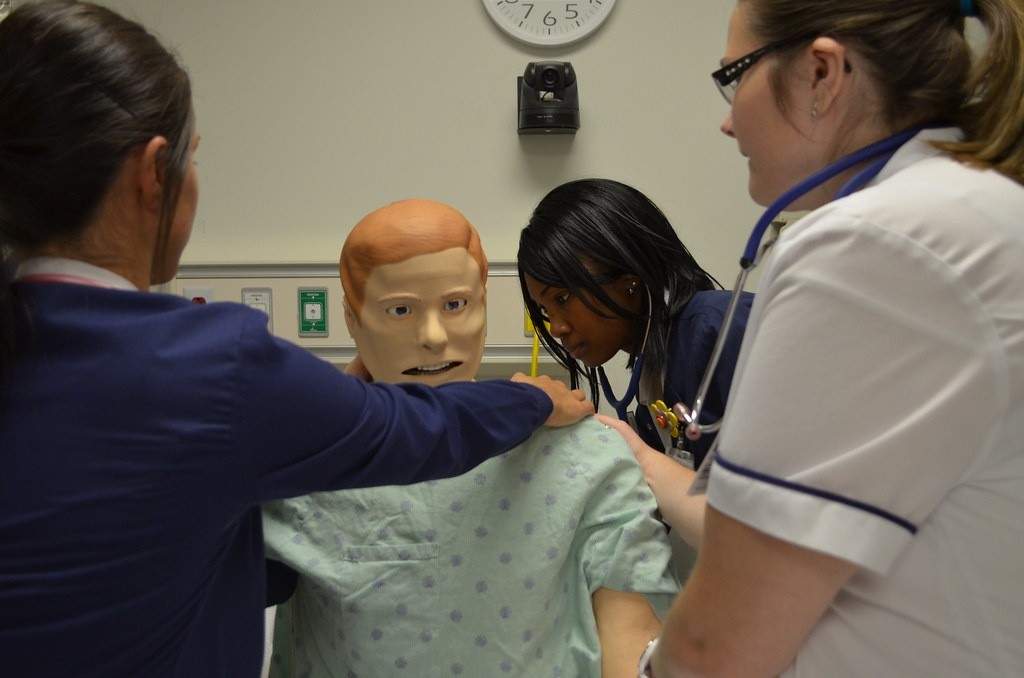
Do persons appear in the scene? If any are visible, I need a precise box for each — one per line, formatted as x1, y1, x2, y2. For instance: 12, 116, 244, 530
0, 0, 594, 678
264, 200, 680, 678
517, 179, 756, 467
595, 0, 1024, 678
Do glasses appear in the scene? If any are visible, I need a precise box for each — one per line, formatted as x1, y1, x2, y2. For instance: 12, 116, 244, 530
712, 29, 852, 107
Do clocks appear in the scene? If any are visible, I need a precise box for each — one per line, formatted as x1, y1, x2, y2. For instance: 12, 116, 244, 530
480, 0, 616, 48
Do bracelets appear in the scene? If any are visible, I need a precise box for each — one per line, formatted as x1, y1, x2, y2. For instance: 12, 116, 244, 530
638, 637, 658, 678
